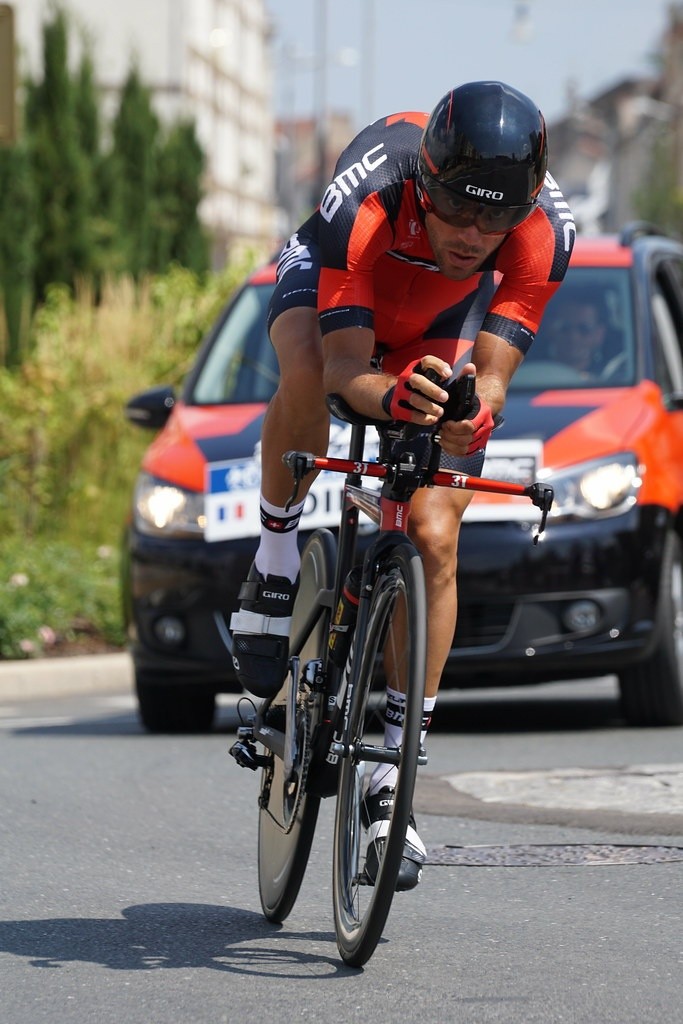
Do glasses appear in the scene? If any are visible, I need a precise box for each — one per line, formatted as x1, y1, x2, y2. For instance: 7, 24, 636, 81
420, 174, 538, 236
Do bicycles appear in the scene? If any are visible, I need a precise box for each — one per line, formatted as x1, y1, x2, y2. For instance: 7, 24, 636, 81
227, 361, 553, 964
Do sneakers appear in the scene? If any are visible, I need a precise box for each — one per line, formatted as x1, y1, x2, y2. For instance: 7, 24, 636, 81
230, 561, 300, 698
360, 783, 427, 893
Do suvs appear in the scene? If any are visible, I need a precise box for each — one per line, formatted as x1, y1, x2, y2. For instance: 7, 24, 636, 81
119, 211, 683, 724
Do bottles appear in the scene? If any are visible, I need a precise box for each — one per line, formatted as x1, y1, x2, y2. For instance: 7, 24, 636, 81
326, 563, 365, 665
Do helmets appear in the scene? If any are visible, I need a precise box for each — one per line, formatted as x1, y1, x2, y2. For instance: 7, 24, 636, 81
418, 80, 549, 216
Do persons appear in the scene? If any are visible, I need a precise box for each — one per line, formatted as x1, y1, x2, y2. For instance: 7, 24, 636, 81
224, 80, 578, 896
539, 283, 627, 384
